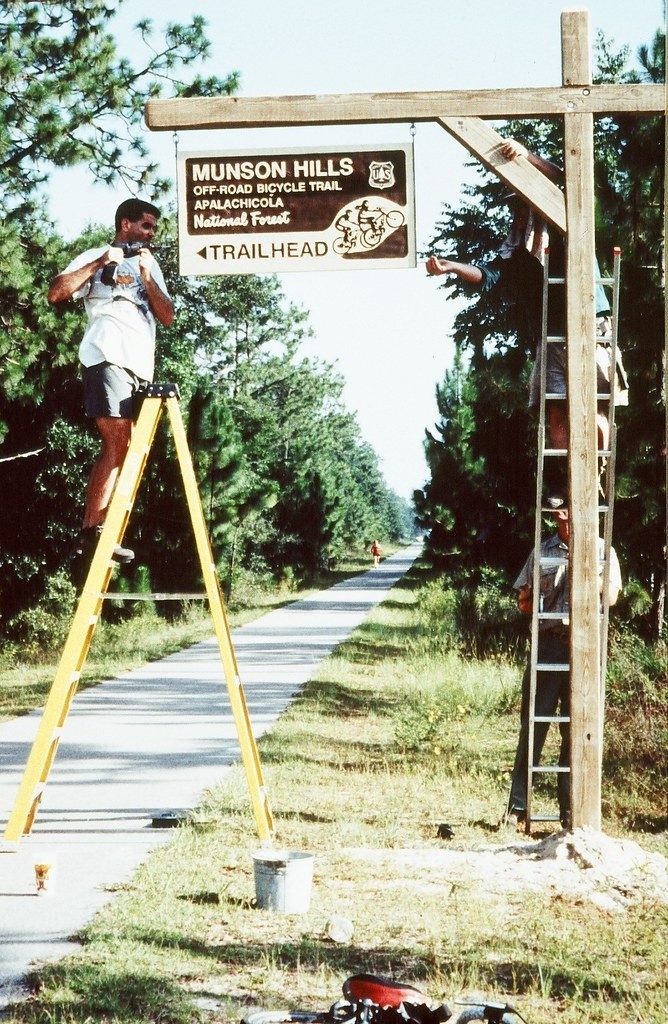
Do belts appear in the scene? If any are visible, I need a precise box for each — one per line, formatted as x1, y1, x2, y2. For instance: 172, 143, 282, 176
542, 631, 569, 644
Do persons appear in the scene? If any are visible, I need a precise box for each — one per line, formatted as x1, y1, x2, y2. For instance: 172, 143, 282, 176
371, 540, 380, 566
47, 198, 175, 565
425, 137, 630, 471
499, 496, 622, 826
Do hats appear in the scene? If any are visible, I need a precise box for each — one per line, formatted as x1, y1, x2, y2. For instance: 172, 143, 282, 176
498, 189, 517, 203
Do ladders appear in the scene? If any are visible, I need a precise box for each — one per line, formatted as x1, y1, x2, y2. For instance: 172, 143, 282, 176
0, 376, 279, 855
525, 248, 624, 839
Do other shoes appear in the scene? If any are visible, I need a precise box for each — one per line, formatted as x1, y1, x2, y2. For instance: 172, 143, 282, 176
559, 810, 571, 828
541, 485, 567, 508
497, 811, 526, 826
78, 524, 134, 564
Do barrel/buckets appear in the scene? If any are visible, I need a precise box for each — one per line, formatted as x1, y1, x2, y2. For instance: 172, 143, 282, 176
252, 850, 315, 913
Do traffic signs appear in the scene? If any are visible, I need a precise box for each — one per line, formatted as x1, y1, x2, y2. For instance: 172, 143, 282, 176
174, 139, 423, 277
